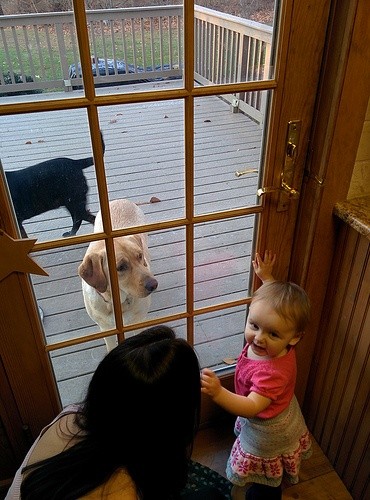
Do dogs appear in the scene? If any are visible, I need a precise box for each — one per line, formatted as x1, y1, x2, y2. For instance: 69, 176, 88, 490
5, 130, 106, 240
77, 199, 158, 353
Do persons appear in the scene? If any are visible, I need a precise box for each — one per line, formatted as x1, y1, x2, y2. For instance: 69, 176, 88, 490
198, 250, 317, 500
0, 324, 239, 500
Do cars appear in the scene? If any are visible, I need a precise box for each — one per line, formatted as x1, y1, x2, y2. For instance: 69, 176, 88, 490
0, 70, 43, 96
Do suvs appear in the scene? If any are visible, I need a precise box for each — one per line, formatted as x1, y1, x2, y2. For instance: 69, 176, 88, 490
68, 58, 183, 91
